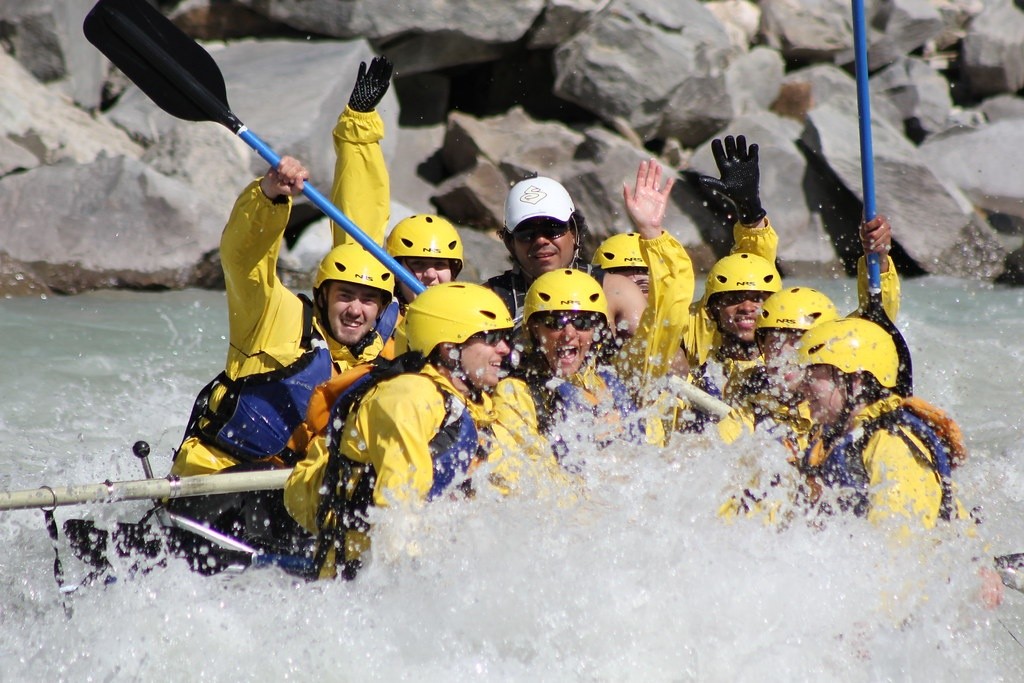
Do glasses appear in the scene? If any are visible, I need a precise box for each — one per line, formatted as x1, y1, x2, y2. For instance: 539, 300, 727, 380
468, 330, 515, 347
512, 218, 572, 242
531, 313, 597, 331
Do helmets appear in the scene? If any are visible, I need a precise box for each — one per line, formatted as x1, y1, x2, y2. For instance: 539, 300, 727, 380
590, 232, 648, 271
785, 317, 898, 389
522, 269, 609, 327
405, 282, 515, 358
703, 252, 783, 307
313, 241, 396, 305
387, 213, 464, 280
754, 285, 837, 344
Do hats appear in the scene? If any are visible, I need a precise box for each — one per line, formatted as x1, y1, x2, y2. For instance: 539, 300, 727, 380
504, 176, 576, 236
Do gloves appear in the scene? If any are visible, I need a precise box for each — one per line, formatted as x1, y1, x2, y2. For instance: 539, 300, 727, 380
698, 134, 767, 224
348, 54, 394, 112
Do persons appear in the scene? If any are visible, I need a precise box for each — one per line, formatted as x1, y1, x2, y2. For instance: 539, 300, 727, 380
282, 54, 900, 579
794, 317, 1004, 640
161, 156, 396, 578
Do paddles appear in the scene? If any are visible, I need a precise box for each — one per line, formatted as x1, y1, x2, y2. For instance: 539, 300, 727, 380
81, 1, 427, 296
668, 375, 1024, 593
1, 465, 295, 510
848, 0, 915, 398
